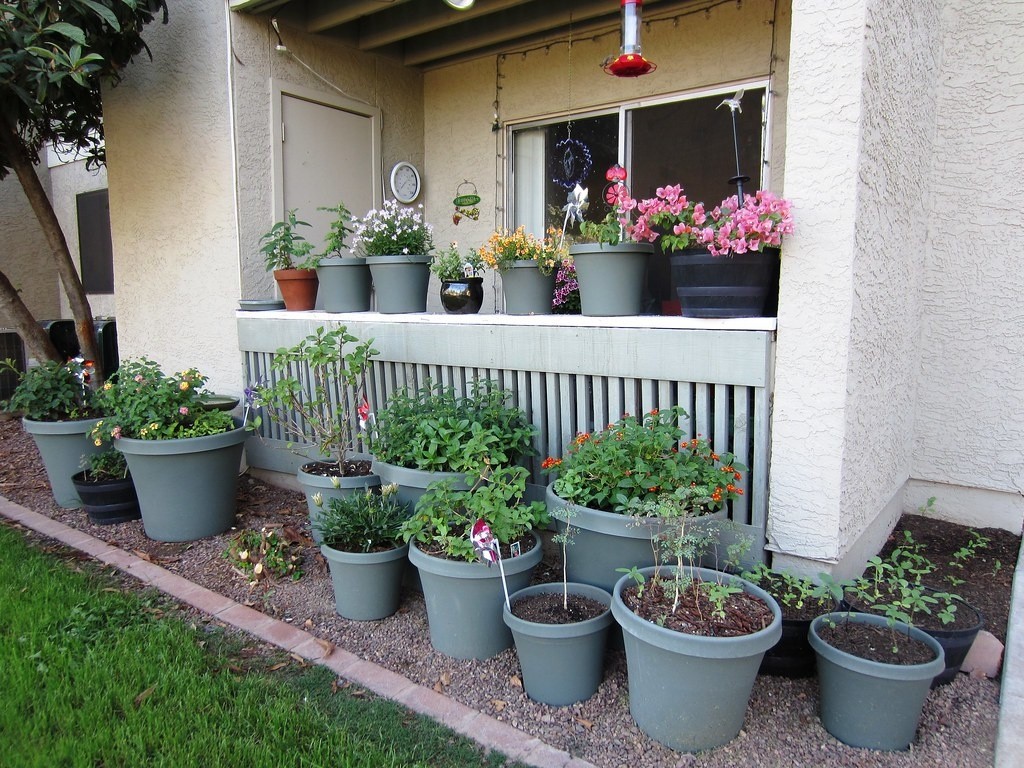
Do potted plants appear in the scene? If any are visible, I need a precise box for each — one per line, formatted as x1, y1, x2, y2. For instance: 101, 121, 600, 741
733, 559, 842, 682
806, 574, 946, 752
393, 418, 551, 659
71, 446, 142, 525
296, 200, 372, 313
569, 199, 656, 315
369, 370, 542, 516
500, 469, 613, 708
841, 552, 982, 690
610, 486, 784, 756
257, 206, 319, 311
242, 324, 390, 545
0, 358, 118, 510
429, 240, 486, 315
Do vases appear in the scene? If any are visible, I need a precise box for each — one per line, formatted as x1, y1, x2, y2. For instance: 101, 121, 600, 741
318, 542, 408, 623
365, 256, 435, 313
112, 416, 252, 544
500, 259, 560, 315
544, 478, 729, 596
668, 246, 781, 318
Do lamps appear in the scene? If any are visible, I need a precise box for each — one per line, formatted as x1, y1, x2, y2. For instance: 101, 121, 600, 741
601, 0, 657, 80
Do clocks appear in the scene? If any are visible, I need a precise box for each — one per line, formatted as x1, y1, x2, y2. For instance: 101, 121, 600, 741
391, 161, 421, 204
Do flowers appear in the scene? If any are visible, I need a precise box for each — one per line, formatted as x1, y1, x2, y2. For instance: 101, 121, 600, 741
348, 199, 436, 256
615, 182, 795, 260
84, 354, 236, 447
302, 473, 412, 552
540, 404, 751, 521
552, 259, 582, 315
477, 225, 573, 277
